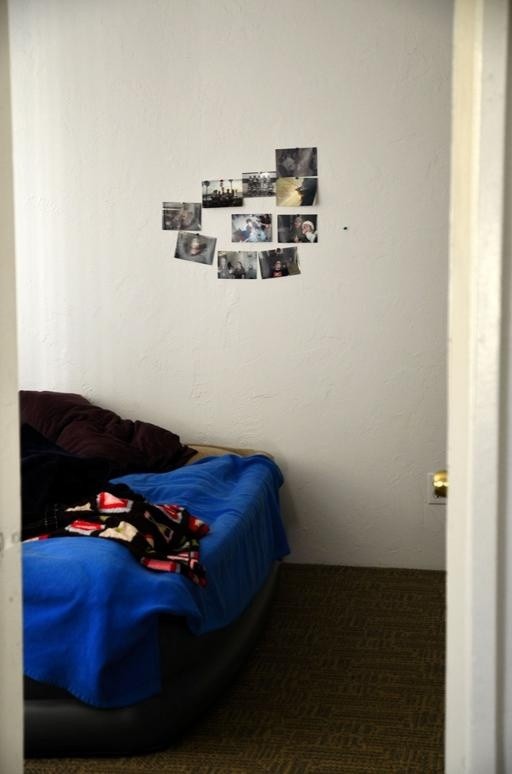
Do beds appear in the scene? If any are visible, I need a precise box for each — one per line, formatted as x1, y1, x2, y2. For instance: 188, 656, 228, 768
21, 443, 284, 760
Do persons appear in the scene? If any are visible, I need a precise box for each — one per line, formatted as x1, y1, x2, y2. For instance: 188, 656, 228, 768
161, 144, 320, 280
